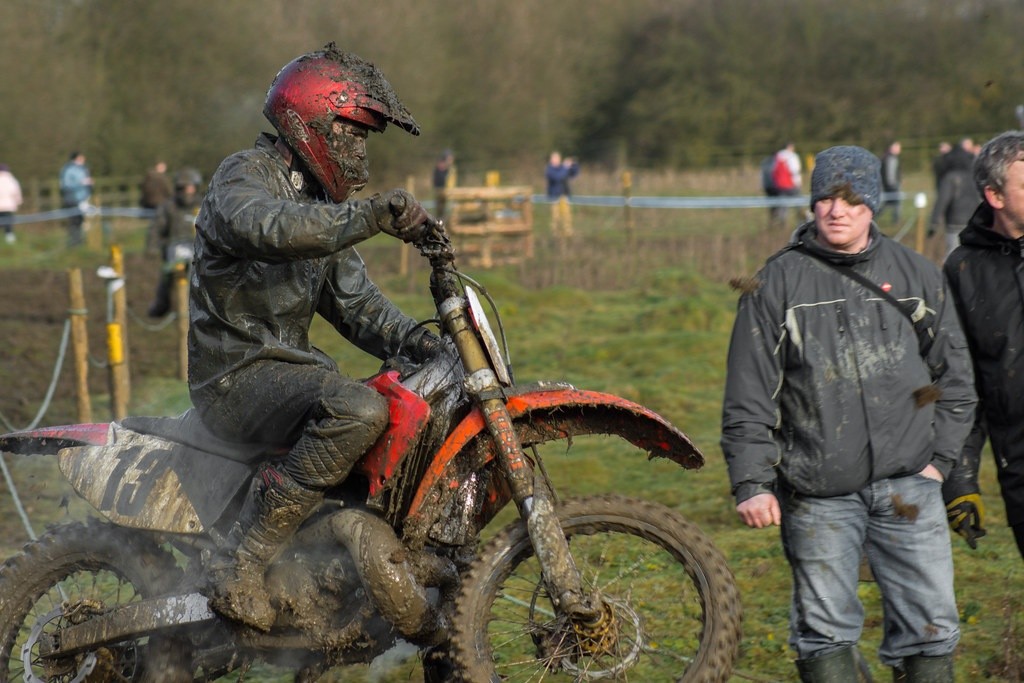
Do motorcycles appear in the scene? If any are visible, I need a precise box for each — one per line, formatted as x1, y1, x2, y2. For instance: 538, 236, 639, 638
0, 189, 749, 683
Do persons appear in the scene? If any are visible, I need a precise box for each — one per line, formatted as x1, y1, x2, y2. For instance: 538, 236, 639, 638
928, 139, 982, 251
718, 146, 979, 683
187, 50, 441, 633
761, 142, 813, 227
434, 153, 447, 218
941, 130, 1024, 560
545, 151, 579, 230
60, 152, 94, 246
0, 162, 24, 241
146, 165, 203, 318
141, 161, 169, 208
880, 141, 902, 223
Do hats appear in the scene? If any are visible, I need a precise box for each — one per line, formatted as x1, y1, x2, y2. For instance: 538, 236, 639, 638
811, 146, 883, 216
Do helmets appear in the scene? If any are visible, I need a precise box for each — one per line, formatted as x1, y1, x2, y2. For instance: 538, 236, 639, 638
263, 49, 421, 205
173, 170, 203, 207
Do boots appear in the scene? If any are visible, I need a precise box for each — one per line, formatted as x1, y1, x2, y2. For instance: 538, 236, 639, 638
795, 640, 860, 683
893, 652, 954, 683
184, 459, 325, 629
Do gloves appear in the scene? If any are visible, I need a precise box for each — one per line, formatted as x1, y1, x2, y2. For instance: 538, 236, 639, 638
941, 477, 987, 550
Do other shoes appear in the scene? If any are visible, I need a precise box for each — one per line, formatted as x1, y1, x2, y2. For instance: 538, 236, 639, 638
149, 301, 171, 318
